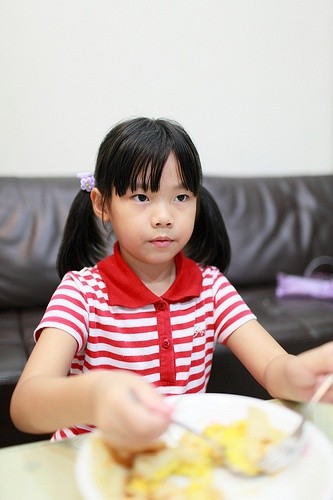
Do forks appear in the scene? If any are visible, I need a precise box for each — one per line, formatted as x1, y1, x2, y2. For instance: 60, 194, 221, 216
255, 371, 333, 475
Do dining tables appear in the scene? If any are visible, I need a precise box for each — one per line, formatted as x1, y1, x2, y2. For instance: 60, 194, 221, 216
0, 398, 333, 499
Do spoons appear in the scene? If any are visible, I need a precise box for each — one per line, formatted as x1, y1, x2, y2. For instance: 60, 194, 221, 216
166, 414, 257, 476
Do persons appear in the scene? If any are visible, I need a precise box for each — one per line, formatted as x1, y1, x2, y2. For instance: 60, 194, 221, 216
10, 117, 333, 443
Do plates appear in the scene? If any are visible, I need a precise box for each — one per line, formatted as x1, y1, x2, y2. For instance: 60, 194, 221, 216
76, 393, 333, 500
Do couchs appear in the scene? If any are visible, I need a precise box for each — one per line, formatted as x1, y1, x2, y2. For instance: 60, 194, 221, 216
1, 173, 333, 452
